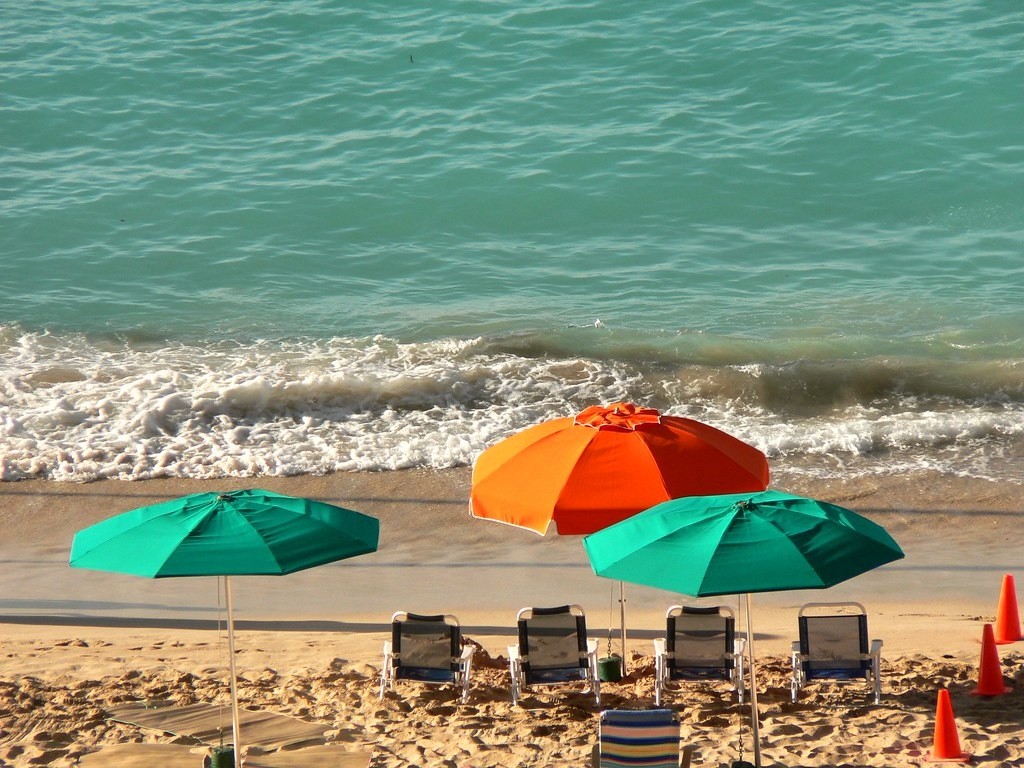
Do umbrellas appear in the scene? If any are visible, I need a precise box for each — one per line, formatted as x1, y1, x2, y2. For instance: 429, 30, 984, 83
582, 490, 904, 767
68, 489, 380, 768
468, 403, 770, 677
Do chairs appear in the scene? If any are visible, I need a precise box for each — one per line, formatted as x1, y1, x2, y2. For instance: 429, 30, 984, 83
381, 612, 477, 705
790, 602, 884, 705
654, 605, 746, 710
508, 604, 603, 706
590, 708, 700, 768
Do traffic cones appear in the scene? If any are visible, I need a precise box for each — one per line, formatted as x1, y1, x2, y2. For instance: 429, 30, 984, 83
977, 573, 1024, 644
922, 689, 971, 763
968, 625, 1015, 696
